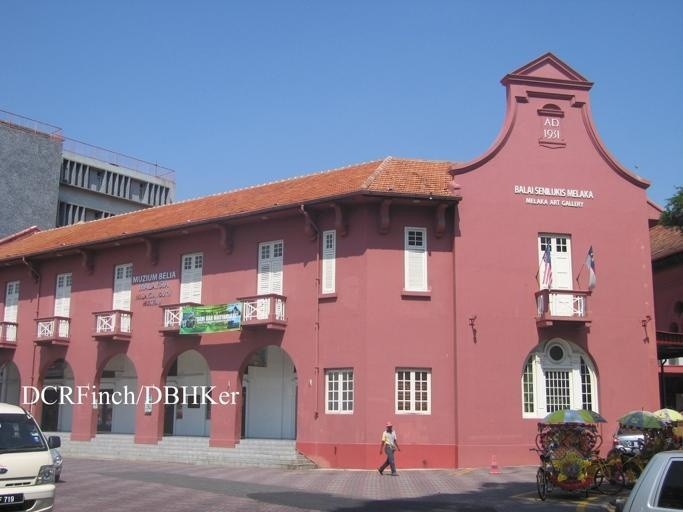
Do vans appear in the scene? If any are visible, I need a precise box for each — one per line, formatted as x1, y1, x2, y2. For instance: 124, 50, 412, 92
0, 401, 54, 512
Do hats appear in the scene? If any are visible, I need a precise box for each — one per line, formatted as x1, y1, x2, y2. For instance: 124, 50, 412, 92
385, 421, 392, 426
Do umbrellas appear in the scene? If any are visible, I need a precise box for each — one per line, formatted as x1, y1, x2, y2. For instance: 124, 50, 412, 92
616, 408, 683, 428
543, 410, 606, 425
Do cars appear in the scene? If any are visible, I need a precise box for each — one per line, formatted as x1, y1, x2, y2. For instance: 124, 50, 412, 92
622, 451, 683, 511
54, 450, 61, 480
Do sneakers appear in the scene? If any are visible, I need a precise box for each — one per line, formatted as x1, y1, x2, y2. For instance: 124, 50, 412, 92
377, 468, 399, 475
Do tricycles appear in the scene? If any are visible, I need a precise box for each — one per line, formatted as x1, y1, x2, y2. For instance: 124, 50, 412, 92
530, 408, 683, 501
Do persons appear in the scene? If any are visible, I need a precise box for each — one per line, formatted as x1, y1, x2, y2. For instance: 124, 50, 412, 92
377, 422, 401, 476
617, 423, 683, 461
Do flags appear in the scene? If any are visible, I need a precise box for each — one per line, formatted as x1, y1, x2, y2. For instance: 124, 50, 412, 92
587, 254, 595, 290
542, 246, 552, 292
